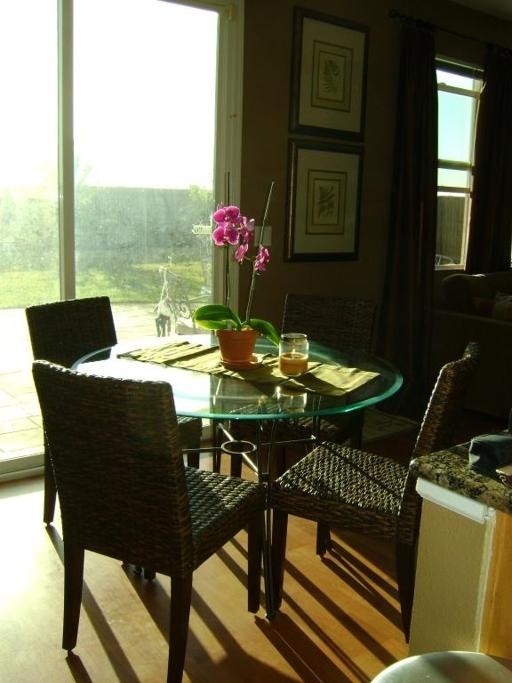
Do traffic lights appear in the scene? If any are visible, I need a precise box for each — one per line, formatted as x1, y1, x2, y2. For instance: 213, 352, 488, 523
441, 271, 512, 321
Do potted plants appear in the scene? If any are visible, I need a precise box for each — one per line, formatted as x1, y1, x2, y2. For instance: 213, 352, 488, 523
284, 138, 365, 262
289, 6, 370, 142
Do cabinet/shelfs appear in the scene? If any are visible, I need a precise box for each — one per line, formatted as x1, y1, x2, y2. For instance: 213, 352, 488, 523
278, 332, 309, 377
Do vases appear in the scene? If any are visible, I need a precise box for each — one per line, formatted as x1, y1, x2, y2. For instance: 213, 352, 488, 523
191, 182, 280, 347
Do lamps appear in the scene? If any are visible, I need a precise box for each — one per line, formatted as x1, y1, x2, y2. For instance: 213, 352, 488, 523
31, 358, 268, 683
268, 341, 481, 644
438, 295, 512, 417
228, 293, 377, 479
26, 297, 202, 568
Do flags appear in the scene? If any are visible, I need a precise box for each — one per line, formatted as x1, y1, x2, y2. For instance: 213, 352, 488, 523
215, 327, 259, 371
